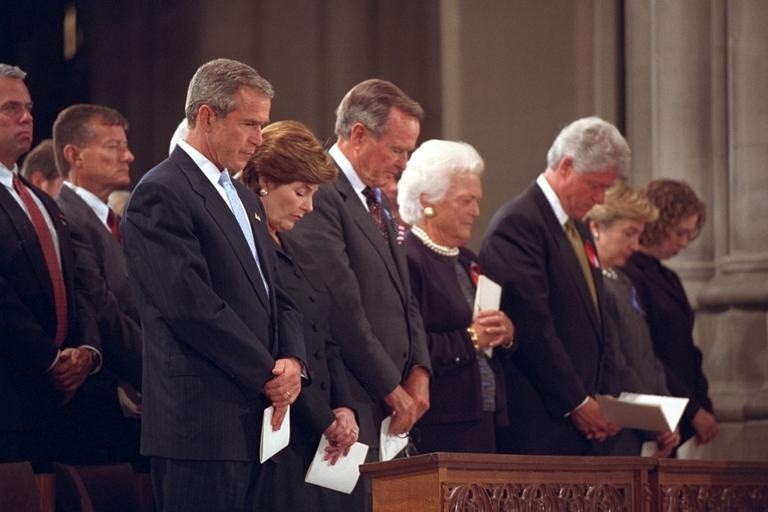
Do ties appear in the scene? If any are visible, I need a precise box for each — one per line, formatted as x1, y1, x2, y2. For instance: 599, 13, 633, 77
360, 185, 390, 255
10, 172, 70, 349
217, 167, 272, 302
565, 219, 601, 319
105, 206, 121, 249
